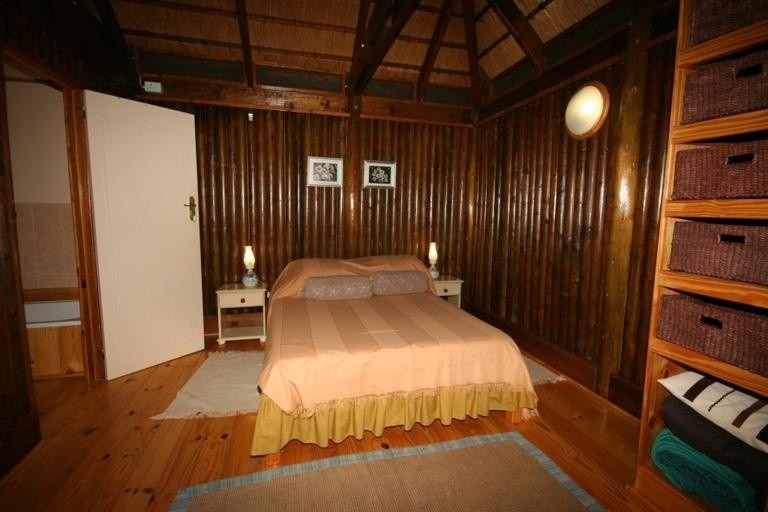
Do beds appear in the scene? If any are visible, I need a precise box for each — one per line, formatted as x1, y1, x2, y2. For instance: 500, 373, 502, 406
257, 253, 524, 463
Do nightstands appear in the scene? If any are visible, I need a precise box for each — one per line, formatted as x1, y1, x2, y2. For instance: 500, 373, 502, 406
214, 282, 267, 344
433, 275, 464, 308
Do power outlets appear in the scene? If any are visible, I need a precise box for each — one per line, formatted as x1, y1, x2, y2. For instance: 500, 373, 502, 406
144, 81, 162, 94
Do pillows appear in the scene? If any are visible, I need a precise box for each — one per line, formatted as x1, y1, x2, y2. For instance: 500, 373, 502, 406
302, 274, 374, 300
656, 369, 767, 453
374, 271, 430, 295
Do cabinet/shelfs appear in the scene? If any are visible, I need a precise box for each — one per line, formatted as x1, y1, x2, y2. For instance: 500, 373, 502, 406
634, 0, 767, 508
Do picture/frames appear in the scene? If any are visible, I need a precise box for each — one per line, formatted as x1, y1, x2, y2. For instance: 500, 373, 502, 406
361, 160, 397, 189
305, 156, 343, 188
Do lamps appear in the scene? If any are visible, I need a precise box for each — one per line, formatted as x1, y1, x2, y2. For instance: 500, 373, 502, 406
428, 242, 439, 279
563, 79, 611, 140
243, 245, 259, 288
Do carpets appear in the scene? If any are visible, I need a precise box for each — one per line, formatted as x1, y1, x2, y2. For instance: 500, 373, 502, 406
523, 352, 569, 386
169, 427, 606, 509
150, 347, 260, 420
